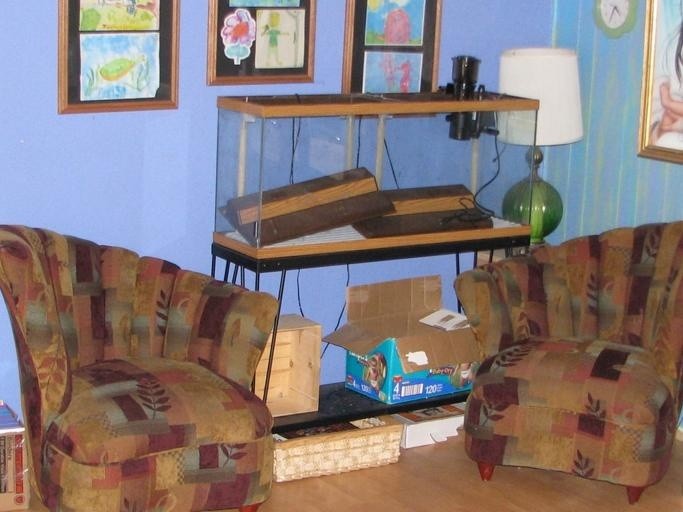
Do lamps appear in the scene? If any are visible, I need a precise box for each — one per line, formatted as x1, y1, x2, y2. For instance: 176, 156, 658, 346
500, 48, 583, 242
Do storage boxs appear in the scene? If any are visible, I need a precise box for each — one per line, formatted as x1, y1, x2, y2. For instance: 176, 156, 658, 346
0, 401, 30, 512
272, 415, 403, 483
323, 274, 482, 406
252, 313, 322, 417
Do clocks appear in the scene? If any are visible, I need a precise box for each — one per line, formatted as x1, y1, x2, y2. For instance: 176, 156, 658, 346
594, 0, 638, 38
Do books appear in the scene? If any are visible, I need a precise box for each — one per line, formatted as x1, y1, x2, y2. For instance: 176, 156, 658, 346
390, 401, 466, 450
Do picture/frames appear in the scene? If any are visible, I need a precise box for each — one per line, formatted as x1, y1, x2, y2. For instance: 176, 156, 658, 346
638, 0, 683, 163
207, 0, 318, 86
345, 0, 441, 119
58, 0, 180, 114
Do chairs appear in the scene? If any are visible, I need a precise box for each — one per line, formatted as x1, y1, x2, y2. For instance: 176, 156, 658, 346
0, 223, 279, 512
454, 220, 683, 504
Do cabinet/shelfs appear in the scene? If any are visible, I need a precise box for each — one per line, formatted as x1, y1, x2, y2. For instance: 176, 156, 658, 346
219, 93, 539, 430
